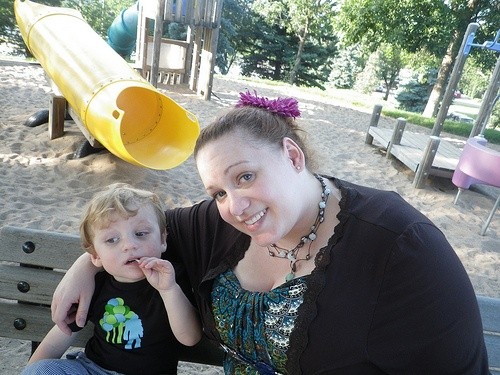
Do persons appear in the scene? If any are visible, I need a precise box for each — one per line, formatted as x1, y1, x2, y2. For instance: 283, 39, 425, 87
51, 87, 492, 375
18, 183, 202, 375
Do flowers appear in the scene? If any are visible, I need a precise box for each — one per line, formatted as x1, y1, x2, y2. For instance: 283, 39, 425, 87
236, 89, 302, 121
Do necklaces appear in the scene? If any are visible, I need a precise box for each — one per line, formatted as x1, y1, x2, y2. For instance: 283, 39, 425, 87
266, 174, 330, 282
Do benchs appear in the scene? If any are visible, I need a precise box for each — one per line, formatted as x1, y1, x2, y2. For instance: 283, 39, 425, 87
0, 226, 500, 375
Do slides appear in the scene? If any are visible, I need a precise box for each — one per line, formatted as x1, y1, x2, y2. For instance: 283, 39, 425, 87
107, 4, 138, 56
13, 0, 201, 171
